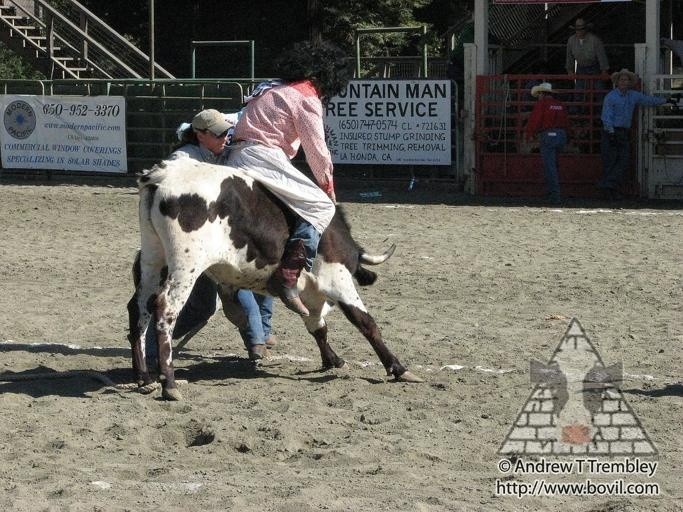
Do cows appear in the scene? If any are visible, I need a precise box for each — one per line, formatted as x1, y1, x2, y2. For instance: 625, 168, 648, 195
125, 157, 427, 402
480, 135, 601, 154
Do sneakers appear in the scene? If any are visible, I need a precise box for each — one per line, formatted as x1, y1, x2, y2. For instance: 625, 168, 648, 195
248, 335, 277, 359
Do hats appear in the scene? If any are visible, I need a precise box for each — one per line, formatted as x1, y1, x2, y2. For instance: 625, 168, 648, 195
191, 108, 236, 136
568, 18, 595, 30
531, 82, 560, 97
611, 69, 639, 86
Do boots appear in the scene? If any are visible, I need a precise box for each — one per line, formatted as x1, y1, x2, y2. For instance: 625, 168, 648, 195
268, 238, 309, 317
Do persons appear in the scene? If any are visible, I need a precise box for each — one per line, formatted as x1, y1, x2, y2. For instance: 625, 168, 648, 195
523, 83, 567, 203
600, 69, 676, 199
567, 18, 611, 139
127, 39, 353, 379
